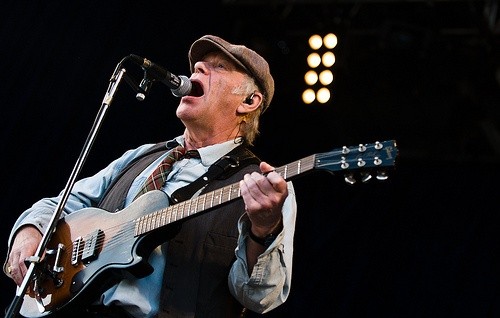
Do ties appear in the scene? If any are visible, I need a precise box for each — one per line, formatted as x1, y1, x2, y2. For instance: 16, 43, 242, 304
132, 145, 201, 202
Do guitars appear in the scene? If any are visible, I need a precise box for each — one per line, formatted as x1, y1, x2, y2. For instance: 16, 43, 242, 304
15, 140, 398, 318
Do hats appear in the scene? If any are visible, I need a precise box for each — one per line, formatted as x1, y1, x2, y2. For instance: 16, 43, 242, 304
188, 35, 274, 115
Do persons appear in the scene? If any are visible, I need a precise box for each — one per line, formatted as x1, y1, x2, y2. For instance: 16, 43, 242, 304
1, 34, 298, 318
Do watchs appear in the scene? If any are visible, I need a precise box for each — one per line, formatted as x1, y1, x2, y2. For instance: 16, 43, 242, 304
248, 223, 283, 245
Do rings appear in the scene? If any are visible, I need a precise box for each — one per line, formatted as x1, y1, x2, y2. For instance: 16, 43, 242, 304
7, 267, 12, 273
